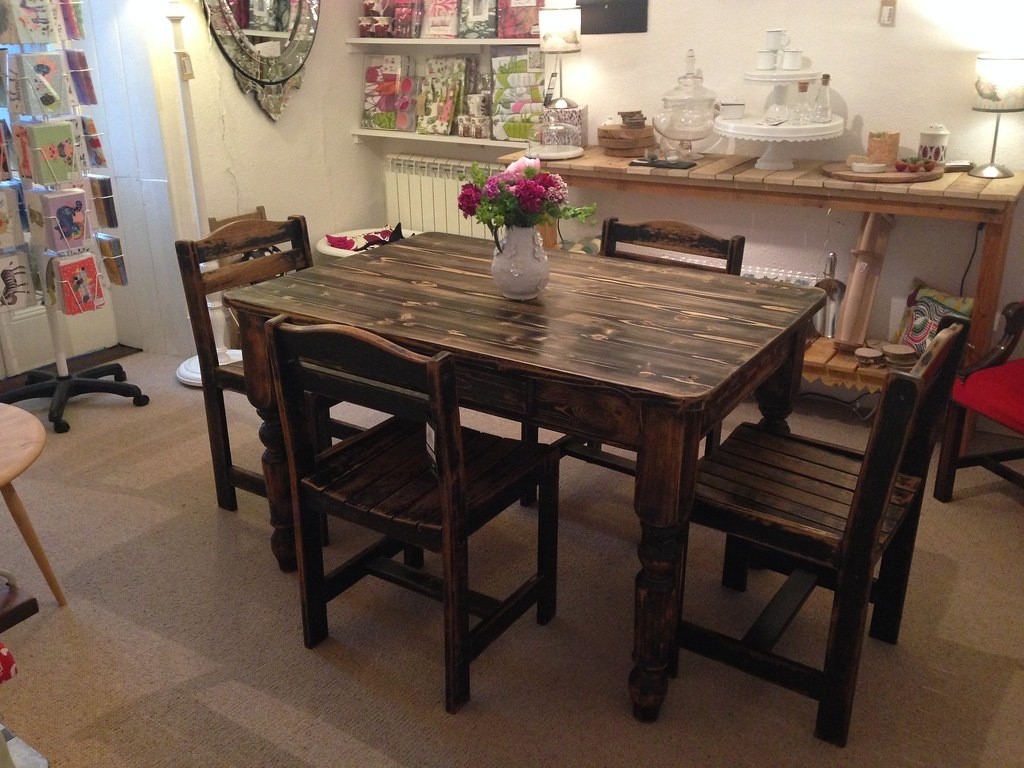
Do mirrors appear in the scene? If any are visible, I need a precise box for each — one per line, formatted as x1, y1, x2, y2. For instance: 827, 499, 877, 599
199, 0, 321, 122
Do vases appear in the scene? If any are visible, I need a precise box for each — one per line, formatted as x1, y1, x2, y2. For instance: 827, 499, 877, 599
491, 225, 550, 300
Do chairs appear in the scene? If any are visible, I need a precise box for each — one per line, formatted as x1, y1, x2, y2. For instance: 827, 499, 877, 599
174, 215, 369, 545
679, 324, 967, 749
207, 205, 268, 348
264, 312, 562, 713
521, 217, 747, 507
933, 302, 1024, 502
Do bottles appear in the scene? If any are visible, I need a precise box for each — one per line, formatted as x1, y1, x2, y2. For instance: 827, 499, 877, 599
812, 74, 831, 123
789, 81, 812, 126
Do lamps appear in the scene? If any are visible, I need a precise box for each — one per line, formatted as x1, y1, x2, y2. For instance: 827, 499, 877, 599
968, 54, 1024, 180
539, 5, 581, 109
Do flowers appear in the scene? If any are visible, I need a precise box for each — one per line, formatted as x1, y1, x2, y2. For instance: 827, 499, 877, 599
457, 157, 597, 228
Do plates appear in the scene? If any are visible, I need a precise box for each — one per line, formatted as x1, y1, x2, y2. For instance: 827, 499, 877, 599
852, 162, 886, 173
821, 160, 944, 183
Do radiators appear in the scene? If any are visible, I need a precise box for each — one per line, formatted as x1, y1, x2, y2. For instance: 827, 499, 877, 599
384, 153, 508, 241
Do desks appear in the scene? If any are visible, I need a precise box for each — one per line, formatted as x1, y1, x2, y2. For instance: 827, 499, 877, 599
498, 144, 1024, 456
226, 228, 830, 722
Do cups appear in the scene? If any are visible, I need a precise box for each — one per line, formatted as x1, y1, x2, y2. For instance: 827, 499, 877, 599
765, 29, 790, 50
918, 122, 950, 167
756, 49, 783, 70
779, 48, 802, 69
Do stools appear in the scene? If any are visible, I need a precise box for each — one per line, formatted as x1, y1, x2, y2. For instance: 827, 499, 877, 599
0, 403, 66, 608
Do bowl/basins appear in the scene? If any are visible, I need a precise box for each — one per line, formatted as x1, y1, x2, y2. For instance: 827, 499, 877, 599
855, 339, 918, 370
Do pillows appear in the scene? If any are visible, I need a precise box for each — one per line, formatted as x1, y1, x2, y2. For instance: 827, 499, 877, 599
889, 278, 975, 358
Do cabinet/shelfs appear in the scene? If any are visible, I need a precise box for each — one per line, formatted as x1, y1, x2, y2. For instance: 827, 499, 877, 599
345, 37, 541, 149
713, 69, 845, 171
